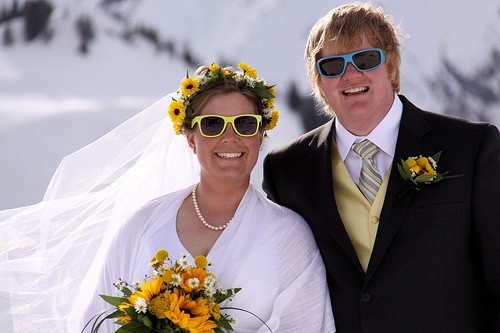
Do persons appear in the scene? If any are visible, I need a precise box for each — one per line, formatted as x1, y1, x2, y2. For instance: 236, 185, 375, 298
261, 3, 500, 333
0, 62, 336, 333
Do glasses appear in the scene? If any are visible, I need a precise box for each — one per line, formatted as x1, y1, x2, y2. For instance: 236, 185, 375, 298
191, 114, 262, 137
317, 48, 385, 78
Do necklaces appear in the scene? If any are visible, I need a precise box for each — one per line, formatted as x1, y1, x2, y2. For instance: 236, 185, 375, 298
191, 183, 234, 230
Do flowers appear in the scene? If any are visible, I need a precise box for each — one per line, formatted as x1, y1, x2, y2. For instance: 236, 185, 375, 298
397, 150, 464, 185
168, 62, 280, 133
80, 249, 273, 333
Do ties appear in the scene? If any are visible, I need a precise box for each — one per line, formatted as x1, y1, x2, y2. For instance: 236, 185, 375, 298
351, 139, 383, 204
